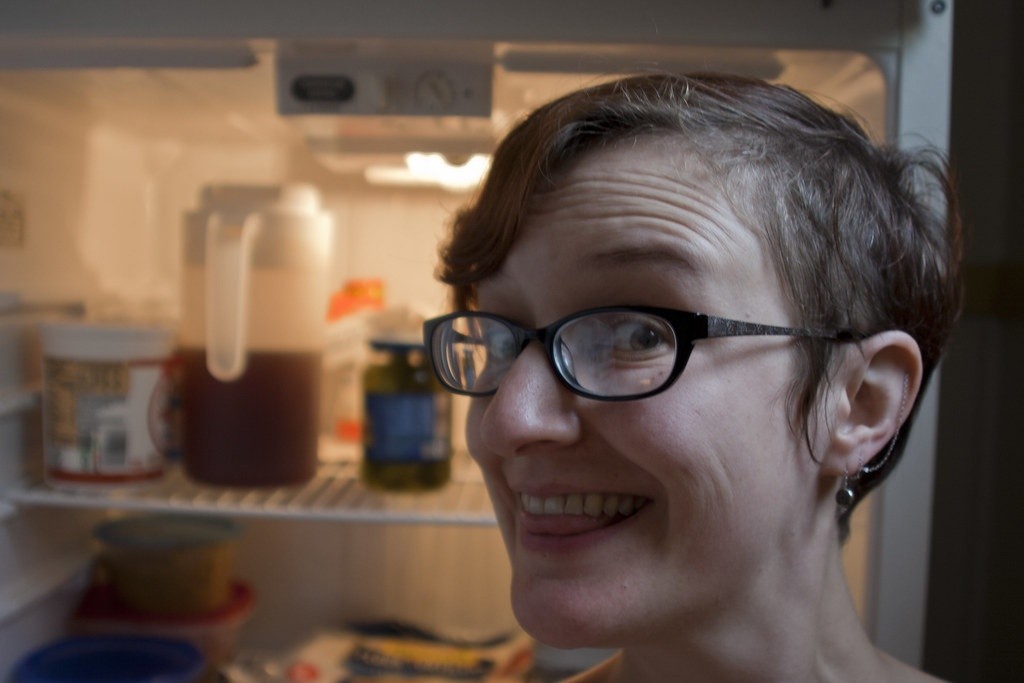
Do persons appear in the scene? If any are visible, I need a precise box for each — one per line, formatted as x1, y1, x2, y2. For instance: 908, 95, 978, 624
435, 75, 968, 683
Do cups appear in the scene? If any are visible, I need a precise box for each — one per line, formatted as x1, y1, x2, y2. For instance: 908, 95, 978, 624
179, 183, 335, 488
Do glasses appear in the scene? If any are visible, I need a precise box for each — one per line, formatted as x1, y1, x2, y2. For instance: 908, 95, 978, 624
424, 303, 871, 402
